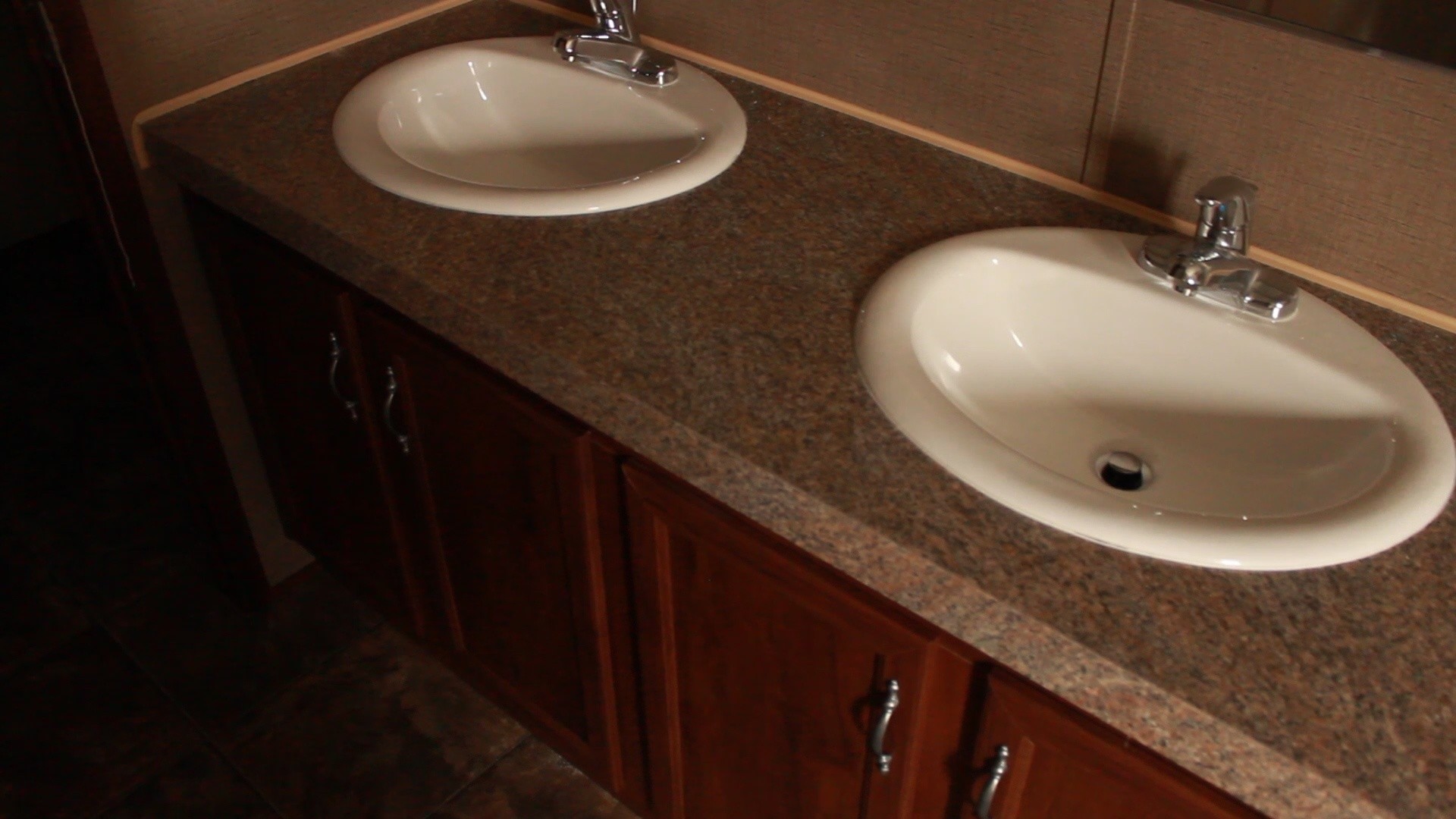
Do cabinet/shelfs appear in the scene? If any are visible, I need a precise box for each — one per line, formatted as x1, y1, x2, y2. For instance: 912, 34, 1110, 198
174, 182, 1280, 818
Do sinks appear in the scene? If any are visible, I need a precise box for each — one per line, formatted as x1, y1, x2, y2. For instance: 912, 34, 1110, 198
849, 224, 1451, 573
328, 34, 751, 217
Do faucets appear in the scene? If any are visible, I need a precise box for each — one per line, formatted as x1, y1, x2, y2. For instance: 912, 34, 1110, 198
1165, 170, 1270, 307
551, 0, 647, 79
